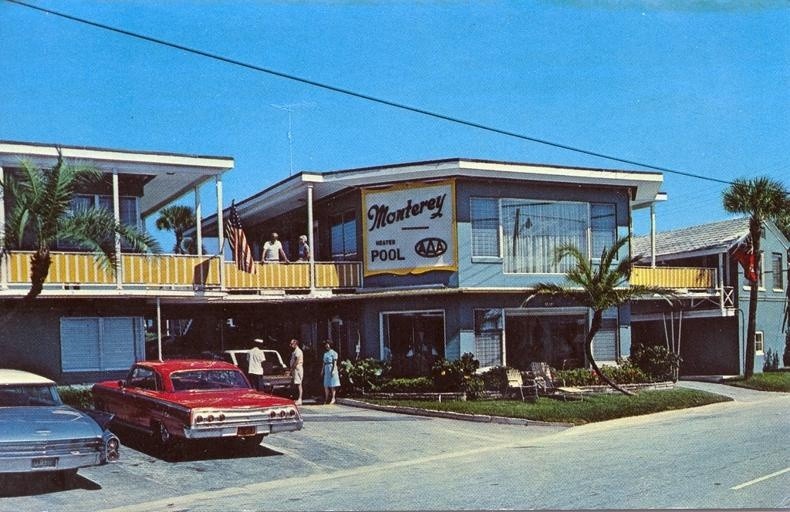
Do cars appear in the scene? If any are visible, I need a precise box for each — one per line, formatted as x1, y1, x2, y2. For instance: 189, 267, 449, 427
220, 346, 293, 394
86, 349, 306, 466
1, 357, 127, 493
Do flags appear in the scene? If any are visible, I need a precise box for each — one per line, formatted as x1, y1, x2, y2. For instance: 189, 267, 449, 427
726, 231, 759, 284
225, 205, 258, 277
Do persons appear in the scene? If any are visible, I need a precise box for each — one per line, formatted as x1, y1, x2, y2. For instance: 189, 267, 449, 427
296, 234, 311, 262
286, 338, 305, 406
320, 338, 341, 405
260, 232, 290, 266
246, 338, 267, 393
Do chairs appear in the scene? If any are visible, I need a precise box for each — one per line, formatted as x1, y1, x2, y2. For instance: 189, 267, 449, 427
503, 361, 594, 403
